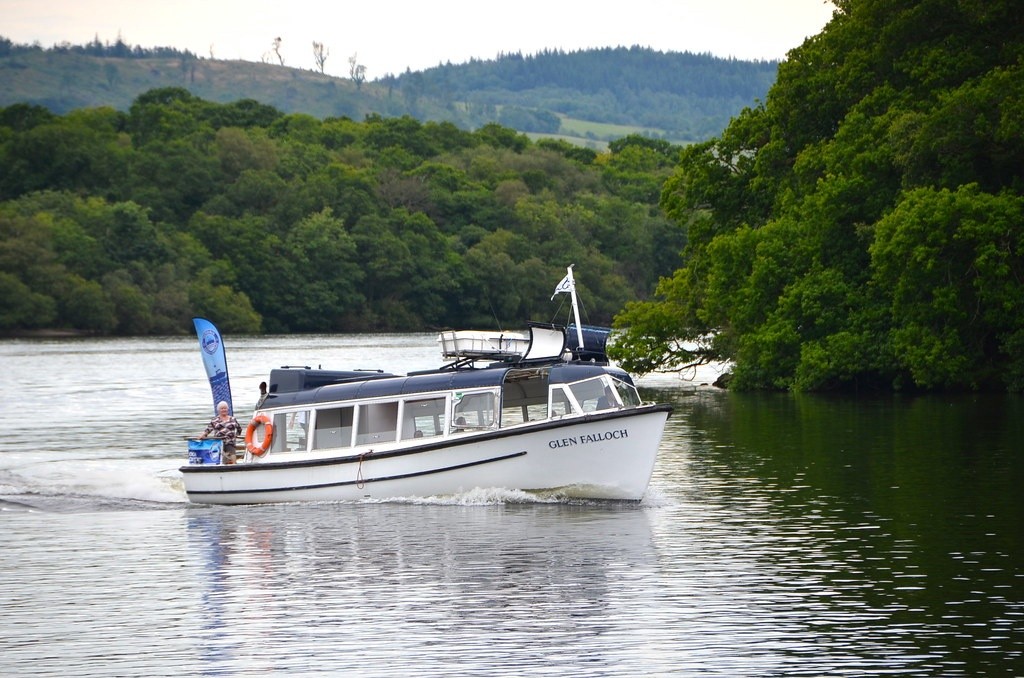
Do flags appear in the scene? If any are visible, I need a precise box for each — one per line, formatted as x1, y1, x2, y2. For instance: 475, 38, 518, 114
550, 272, 577, 302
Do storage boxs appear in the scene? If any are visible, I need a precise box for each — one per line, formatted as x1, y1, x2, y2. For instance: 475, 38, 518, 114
436, 331, 525, 355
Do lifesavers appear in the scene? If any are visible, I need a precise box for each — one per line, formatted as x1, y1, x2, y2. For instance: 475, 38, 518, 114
245, 416, 274, 457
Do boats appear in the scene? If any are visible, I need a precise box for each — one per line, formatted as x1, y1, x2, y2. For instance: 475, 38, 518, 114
179, 256, 676, 511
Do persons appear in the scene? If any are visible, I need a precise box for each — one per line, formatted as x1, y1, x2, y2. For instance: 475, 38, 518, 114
451, 417, 466, 433
196, 400, 243, 465
595, 383, 619, 411
